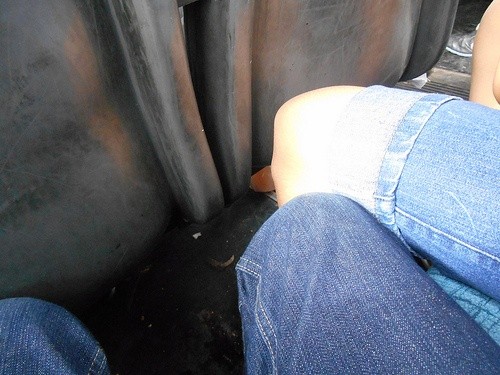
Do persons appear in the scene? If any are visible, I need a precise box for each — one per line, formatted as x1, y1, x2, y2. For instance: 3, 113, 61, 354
1, 192, 500, 375
271, 0, 500, 287
